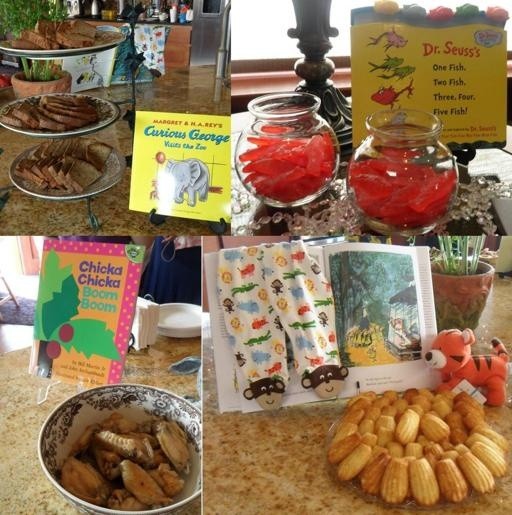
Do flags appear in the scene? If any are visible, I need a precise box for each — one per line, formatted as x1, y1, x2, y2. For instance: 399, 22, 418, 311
422, 325, 508, 409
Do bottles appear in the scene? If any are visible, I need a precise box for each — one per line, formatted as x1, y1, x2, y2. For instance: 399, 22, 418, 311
347, 105, 461, 236
234, 90, 341, 208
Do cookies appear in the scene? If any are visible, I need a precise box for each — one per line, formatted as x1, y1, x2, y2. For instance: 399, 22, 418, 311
327, 385, 510, 507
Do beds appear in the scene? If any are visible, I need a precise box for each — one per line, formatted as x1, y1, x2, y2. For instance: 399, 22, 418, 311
231, 103, 512, 236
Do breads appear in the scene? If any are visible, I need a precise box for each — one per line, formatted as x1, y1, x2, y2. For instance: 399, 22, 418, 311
15, 137, 112, 195
0, 95, 95, 132
11, 18, 98, 49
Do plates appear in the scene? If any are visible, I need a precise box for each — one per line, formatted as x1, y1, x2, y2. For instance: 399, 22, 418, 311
0, 93, 119, 138
0, 30, 127, 60
156, 303, 201, 339
10, 139, 128, 200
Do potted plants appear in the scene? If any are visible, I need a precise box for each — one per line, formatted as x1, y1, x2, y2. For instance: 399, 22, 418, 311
10, 56, 72, 98
428, 236, 495, 332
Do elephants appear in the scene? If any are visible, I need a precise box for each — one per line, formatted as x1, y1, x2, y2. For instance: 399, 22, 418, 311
164, 158, 209, 208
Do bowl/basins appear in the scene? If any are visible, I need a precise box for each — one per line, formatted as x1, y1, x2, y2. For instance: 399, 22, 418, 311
36, 383, 202, 515
